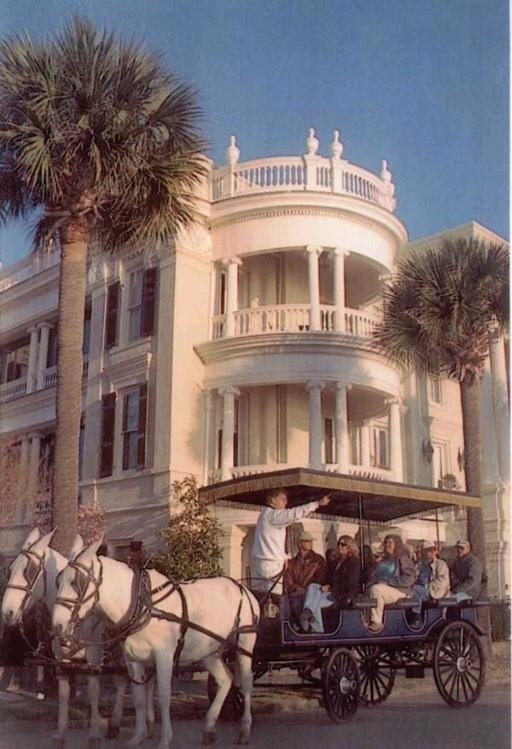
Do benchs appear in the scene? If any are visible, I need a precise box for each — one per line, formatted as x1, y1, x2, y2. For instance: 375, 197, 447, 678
352, 570, 490, 610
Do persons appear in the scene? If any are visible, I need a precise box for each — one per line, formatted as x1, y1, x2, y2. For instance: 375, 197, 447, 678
251, 487, 331, 596
285, 532, 484, 633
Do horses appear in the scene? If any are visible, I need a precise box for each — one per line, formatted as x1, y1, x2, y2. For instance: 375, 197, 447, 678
2, 525, 156, 749
52, 531, 261, 749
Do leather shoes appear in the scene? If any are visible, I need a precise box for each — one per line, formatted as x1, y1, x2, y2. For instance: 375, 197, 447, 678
409, 619, 424, 629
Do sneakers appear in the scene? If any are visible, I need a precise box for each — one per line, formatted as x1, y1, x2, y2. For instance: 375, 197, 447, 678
367, 621, 382, 631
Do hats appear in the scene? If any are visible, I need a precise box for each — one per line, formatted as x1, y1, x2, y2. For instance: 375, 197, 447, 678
423, 539, 438, 549
454, 539, 470, 548
298, 530, 314, 541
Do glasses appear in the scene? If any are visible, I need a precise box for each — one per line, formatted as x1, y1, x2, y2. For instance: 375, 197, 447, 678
337, 541, 347, 547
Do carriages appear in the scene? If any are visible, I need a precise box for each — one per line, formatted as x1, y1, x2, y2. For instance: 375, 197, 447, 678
2, 467, 490, 748
